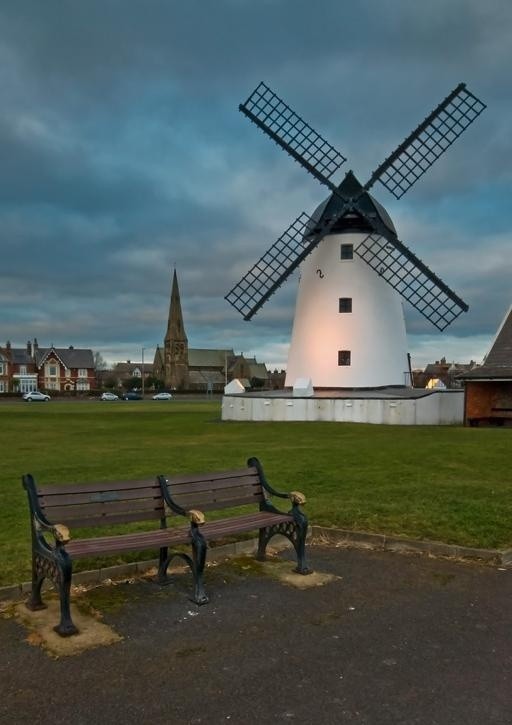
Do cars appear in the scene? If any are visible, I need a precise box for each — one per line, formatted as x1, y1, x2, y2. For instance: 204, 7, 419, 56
100, 393, 119, 401
152, 392, 173, 400
121, 392, 143, 400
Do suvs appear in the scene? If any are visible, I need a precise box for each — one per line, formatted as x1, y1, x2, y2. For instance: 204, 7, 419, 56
22, 391, 51, 402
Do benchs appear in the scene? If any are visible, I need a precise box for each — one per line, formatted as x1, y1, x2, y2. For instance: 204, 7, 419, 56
25, 477, 209, 634
161, 460, 312, 602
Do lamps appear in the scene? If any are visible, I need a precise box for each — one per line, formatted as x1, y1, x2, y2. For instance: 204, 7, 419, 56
294, 376, 313, 397
223, 379, 246, 394
426, 378, 446, 389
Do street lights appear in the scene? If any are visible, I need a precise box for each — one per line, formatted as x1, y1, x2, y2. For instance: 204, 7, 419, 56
141, 347, 147, 399
225, 352, 233, 386
